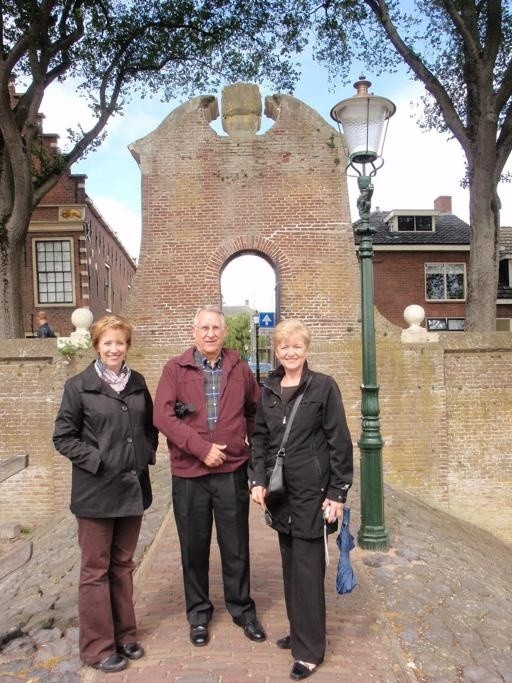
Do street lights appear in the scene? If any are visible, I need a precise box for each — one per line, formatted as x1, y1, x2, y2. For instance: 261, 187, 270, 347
330, 74, 397, 550
252, 310, 261, 387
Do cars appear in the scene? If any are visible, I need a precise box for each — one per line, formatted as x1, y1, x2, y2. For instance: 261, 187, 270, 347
249, 363, 275, 387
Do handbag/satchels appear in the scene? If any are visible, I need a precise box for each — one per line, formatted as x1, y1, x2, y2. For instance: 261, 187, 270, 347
266, 454, 287, 499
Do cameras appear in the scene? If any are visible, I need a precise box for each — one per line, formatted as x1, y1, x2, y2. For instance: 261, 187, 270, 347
322, 504, 336, 523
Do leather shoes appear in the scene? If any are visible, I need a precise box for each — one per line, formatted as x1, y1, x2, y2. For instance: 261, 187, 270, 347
116, 641, 144, 660
291, 663, 313, 680
240, 619, 266, 641
276, 637, 290, 649
190, 623, 209, 646
90, 651, 127, 673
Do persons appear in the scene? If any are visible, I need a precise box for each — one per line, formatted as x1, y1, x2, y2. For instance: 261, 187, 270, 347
245, 317, 355, 683
37, 311, 55, 338
55, 316, 158, 674
149, 304, 272, 648
